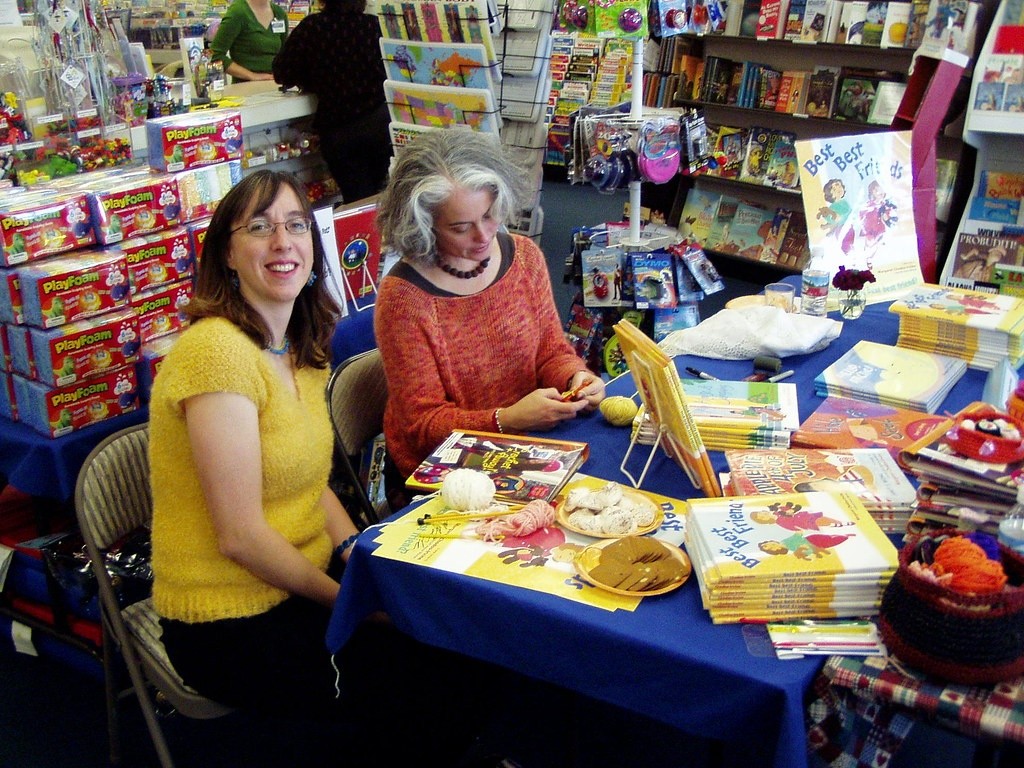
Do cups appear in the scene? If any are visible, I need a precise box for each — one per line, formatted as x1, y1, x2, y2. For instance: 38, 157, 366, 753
763, 284, 796, 312
152, 78, 191, 114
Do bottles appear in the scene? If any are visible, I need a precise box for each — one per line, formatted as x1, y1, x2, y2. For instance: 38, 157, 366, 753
997, 482, 1024, 556
798, 247, 829, 317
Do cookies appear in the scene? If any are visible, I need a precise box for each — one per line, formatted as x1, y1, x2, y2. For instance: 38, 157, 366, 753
588, 535, 690, 592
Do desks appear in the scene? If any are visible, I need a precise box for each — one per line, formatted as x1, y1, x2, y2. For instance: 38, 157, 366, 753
322, 276, 1024, 768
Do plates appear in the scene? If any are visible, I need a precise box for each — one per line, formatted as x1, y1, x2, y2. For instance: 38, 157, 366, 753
573, 538, 691, 597
555, 489, 666, 537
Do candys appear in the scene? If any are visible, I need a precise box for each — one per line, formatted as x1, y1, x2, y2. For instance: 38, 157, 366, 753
0, 105, 133, 187
145, 74, 172, 95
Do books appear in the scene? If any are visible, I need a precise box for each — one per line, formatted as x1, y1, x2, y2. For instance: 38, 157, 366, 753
788, 338, 967, 465
630, 379, 801, 449
718, 445, 919, 533
544, 33, 633, 136
889, 280, 1024, 374
642, 0, 969, 269
683, 488, 903, 625
612, 317, 724, 498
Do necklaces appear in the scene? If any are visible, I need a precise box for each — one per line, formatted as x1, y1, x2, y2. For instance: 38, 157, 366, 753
433, 253, 490, 279
268, 335, 289, 355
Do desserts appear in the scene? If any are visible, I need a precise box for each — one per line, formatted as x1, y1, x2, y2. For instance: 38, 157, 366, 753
564, 481, 655, 535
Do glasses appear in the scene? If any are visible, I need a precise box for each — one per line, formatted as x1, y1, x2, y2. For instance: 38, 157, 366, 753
230, 217, 312, 237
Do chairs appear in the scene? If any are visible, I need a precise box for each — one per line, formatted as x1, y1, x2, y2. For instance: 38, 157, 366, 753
326, 348, 390, 526
75, 422, 236, 768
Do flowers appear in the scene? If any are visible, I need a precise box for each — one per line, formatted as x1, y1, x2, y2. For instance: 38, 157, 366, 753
833, 265, 877, 315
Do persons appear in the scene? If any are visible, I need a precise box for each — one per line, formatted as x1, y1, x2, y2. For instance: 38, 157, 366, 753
210, 0, 289, 80
146, 169, 363, 708
374, 127, 605, 515
270, 0, 395, 205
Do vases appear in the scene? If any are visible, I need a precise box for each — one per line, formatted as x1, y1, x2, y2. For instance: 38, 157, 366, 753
838, 289, 867, 320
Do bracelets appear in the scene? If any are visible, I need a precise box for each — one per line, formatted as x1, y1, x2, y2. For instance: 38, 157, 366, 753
333, 532, 361, 556
495, 407, 505, 434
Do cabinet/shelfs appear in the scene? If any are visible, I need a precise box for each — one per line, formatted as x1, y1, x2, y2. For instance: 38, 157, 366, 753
669, 34, 977, 281
240, 149, 342, 206
1, 295, 378, 768
